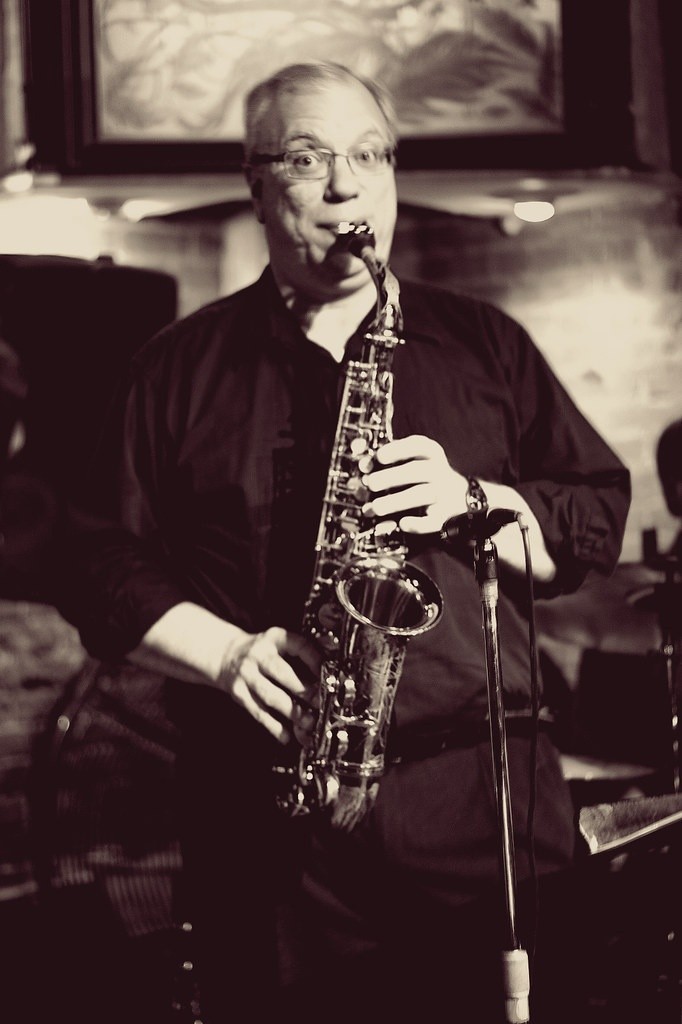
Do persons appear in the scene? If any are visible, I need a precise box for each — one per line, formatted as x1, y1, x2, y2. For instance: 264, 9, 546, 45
51, 63, 632, 1023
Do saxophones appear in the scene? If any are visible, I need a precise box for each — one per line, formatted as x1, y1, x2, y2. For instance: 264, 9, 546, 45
268, 216, 450, 838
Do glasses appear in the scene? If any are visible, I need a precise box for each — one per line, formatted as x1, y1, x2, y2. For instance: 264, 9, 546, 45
248, 148, 396, 179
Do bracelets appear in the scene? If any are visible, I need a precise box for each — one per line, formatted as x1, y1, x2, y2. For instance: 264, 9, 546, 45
467, 474, 488, 513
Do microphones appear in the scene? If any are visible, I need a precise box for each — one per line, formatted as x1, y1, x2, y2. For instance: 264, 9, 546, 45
441, 508, 523, 550
